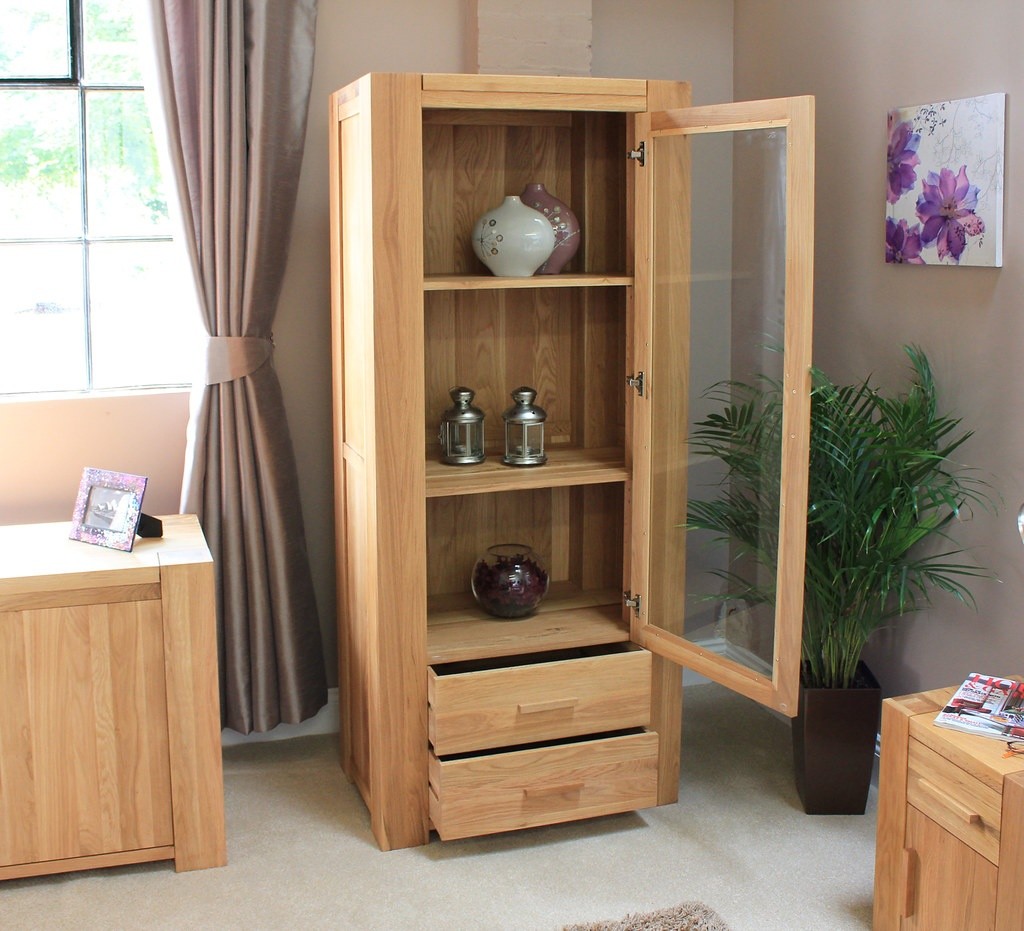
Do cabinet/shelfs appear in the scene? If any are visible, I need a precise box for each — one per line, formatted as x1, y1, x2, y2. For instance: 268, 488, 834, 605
873, 675, 1024, 931
326, 69, 817, 853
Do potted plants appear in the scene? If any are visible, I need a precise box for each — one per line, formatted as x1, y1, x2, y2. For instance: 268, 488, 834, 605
674, 329, 1002, 815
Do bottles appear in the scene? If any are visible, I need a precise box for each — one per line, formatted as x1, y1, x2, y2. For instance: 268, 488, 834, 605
471, 196, 555, 276
470, 543, 550, 619
518, 183, 581, 274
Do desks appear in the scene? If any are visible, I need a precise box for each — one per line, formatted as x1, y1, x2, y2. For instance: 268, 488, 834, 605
0, 514, 226, 880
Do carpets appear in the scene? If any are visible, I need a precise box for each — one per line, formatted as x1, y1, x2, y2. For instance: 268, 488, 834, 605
560, 900, 731, 931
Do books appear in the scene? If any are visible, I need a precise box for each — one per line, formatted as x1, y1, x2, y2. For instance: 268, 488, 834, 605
933, 673, 1024, 743
